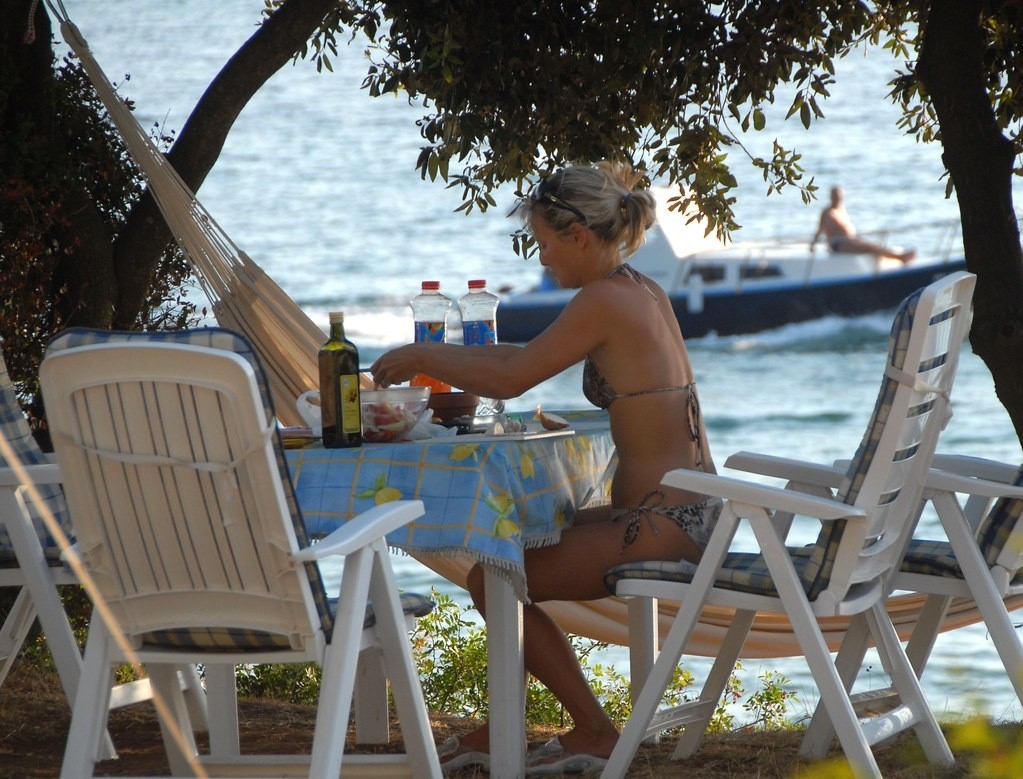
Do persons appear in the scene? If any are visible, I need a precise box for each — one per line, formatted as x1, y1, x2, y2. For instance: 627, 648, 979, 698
810, 186, 917, 263
370, 159, 724, 767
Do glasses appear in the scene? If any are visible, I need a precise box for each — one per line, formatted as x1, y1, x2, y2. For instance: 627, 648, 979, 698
537, 175, 596, 230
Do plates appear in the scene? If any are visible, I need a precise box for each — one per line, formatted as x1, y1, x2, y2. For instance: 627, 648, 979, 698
281, 436, 322, 449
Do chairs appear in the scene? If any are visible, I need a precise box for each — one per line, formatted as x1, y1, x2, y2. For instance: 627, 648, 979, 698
598, 270, 978, 779
0, 327, 444, 779
796, 453, 1022, 779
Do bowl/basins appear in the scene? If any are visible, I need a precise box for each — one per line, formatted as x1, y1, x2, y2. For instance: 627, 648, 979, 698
427, 392, 479, 427
359, 385, 431, 443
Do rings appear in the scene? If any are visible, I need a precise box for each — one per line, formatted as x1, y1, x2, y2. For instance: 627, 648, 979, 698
382, 379, 390, 386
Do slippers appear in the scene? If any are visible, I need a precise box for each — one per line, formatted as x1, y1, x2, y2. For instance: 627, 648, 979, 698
437, 737, 490, 771
524, 735, 609, 774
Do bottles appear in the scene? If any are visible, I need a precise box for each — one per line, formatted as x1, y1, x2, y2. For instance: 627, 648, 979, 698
408, 281, 452, 393
318, 312, 362, 448
456, 279, 506, 417
457, 415, 523, 432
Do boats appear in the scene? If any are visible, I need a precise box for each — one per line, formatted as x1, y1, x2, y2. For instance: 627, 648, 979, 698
444, 216, 1023, 346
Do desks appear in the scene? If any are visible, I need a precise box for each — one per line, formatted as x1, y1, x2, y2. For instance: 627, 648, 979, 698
285, 410, 619, 779
